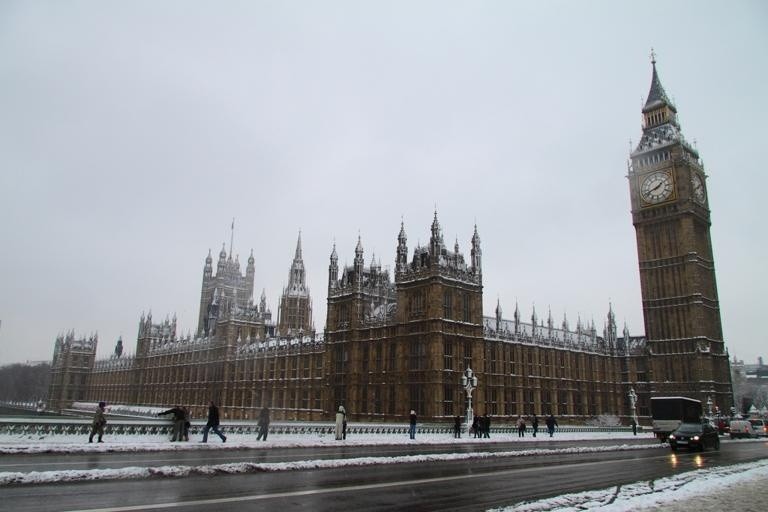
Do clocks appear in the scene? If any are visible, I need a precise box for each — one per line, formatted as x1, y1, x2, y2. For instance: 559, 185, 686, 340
640, 170, 706, 205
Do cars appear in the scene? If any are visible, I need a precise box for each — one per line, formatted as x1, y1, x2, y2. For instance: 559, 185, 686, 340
668, 422, 721, 452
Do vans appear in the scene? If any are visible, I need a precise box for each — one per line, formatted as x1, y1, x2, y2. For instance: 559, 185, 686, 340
749, 418, 768, 437
729, 420, 758, 439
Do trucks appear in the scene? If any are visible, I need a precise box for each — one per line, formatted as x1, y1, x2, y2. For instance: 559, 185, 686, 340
649, 396, 704, 442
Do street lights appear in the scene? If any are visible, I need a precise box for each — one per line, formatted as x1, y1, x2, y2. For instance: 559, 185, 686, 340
462, 365, 479, 436
627, 384, 640, 426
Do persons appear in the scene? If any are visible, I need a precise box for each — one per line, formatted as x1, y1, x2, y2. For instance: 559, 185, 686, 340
409, 410, 417, 439
89, 402, 107, 443
338, 405, 347, 439
155, 405, 191, 442
531, 413, 538, 437
718, 418, 724, 435
546, 414, 558, 437
454, 415, 461, 438
256, 406, 270, 440
472, 412, 491, 438
335, 410, 344, 440
198, 400, 225, 443
632, 420, 636, 435
517, 416, 525, 437
